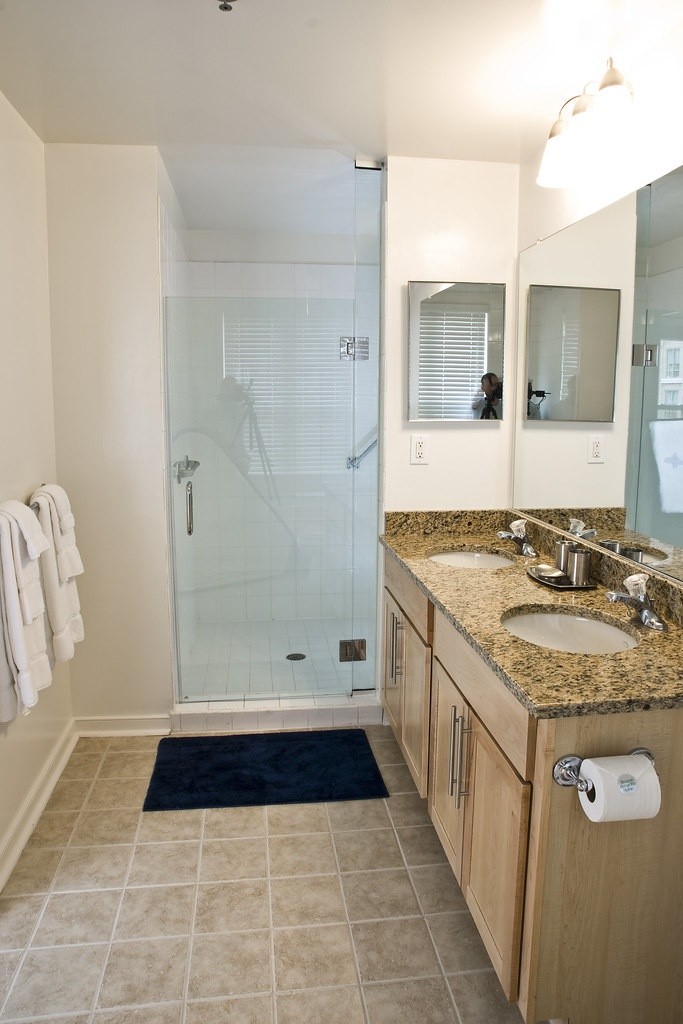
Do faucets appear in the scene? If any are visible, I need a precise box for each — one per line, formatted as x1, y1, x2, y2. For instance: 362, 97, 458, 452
608, 590, 667, 630
580, 529, 599, 541
497, 529, 538, 555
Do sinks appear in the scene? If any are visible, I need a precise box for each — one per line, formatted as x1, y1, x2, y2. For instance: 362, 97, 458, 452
500, 601, 645, 656
422, 543, 516, 571
619, 542, 669, 565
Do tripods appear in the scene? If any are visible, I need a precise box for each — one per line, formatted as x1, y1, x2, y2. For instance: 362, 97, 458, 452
480, 398, 498, 420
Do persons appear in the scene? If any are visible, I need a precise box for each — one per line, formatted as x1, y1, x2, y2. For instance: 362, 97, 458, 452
472, 373, 503, 420
527, 400, 542, 420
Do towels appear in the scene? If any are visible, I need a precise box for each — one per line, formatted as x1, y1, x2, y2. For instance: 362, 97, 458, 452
0, 481, 85, 726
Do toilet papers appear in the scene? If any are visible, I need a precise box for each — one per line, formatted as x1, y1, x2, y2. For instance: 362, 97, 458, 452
576, 754, 664, 826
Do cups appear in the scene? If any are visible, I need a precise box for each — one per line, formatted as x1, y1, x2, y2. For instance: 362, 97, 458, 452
599, 538, 619, 554
567, 549, 591, 584
556, 539, 577, 583
621, 547, 643, 563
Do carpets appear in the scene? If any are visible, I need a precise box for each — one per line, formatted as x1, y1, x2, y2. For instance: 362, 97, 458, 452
142, 727, 390, 812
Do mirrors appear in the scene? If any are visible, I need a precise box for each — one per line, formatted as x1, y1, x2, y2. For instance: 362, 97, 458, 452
508, 164, 682, 590
525, 284, 622, 423
408, 278, 506, 421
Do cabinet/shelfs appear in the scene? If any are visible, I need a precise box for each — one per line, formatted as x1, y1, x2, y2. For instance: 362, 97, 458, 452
426, 604, 683, 1024
379, 549, 436, 806
